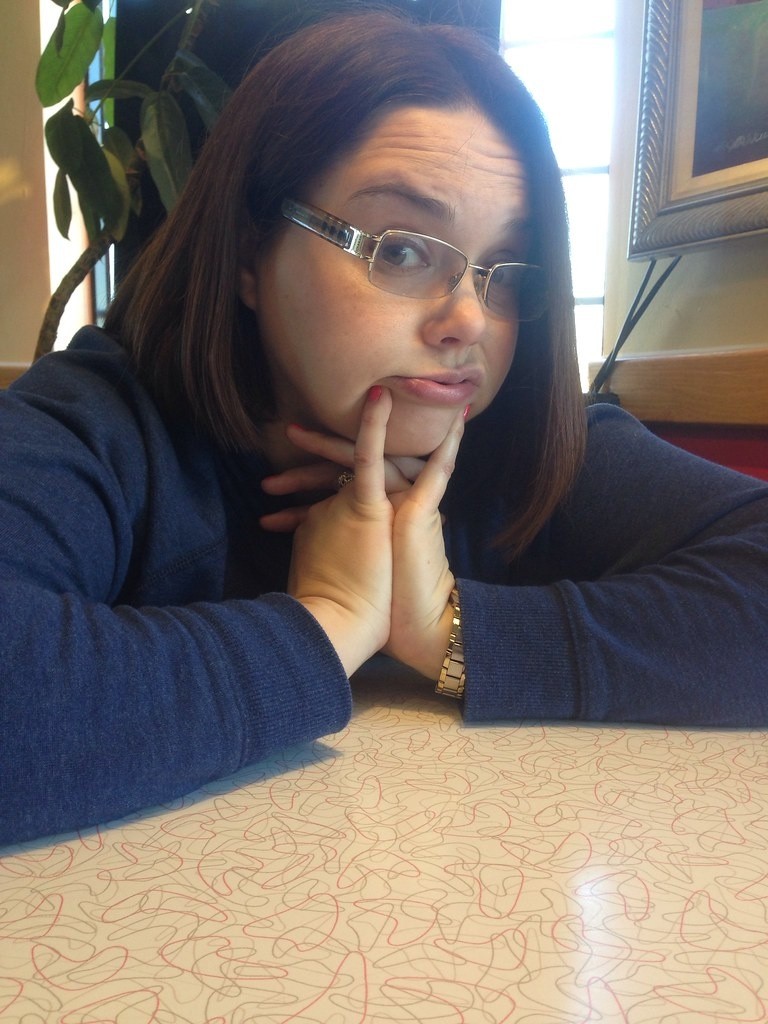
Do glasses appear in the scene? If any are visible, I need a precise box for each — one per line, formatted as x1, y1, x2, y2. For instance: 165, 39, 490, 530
279, 198, 549, 323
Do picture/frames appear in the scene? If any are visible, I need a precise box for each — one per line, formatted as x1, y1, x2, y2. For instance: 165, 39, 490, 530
625, 0, 768, 262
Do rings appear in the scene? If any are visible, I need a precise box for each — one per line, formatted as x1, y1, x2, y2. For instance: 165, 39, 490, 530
338, 471, 354, 488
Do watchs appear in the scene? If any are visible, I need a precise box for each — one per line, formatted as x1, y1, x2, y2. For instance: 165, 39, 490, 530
434, 587, 466, 699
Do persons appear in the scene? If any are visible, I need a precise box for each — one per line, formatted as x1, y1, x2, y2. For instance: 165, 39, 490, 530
1, 14, 768, 853
